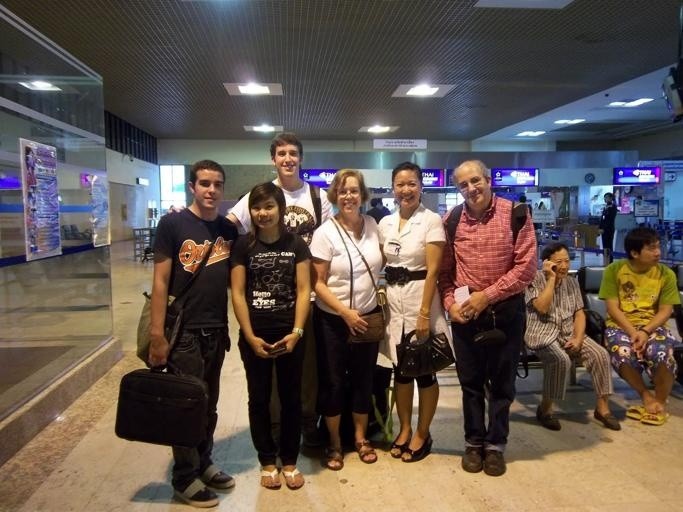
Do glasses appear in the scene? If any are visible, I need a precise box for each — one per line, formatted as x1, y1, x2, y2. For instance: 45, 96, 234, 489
336, 189, 361, 200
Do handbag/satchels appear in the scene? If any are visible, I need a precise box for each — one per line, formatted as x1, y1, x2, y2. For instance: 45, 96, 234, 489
134, 290, 183, 365
115, 363, 212, 448
349, 310, 385, 343
393, 327, 455, 379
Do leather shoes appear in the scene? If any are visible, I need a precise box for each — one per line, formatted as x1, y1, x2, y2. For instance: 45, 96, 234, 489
535, 406, 562, 431
594, 407, 621, 432
460, 441, 484, 472
483, 447, 506, 476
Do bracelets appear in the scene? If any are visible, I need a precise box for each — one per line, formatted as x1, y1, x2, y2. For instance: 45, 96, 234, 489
289, 327, 304, 338
418, 314, 430, 320
638, 328, 651, 338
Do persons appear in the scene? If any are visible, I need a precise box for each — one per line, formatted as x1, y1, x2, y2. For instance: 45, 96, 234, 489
596, 227, 681, 426
306, 167, 385, 471
597, 193, 616, 265
378, 160, 448, 462
522, 241, 621, 431
431, 159, 539, 475
146, 160, 240, 507
363, 198, 391, 223
225, 181, 315, 490
587, 188, 623, 219
512, 195, 547, 217
225, 129, 334, 462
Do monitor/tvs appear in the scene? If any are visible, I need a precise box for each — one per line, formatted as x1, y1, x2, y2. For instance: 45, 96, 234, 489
659, 77, 683, 119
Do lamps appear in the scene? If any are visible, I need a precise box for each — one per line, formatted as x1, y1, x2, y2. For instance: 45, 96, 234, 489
126, 151, 134, 162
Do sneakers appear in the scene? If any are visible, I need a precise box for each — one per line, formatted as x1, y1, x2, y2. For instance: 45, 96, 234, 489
201, 462, 235, 489
171, 476, 219, 507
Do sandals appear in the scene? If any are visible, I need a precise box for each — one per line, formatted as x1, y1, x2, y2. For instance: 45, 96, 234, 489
390, 428, 412, 459
403, 432, 432, 463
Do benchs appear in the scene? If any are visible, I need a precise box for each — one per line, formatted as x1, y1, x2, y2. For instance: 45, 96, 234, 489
430, 353, 588, 396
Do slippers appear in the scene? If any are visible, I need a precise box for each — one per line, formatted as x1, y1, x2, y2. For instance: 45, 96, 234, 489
627, 405, 644, 420
354, 439, 378, 462
282, 465, 306, 490
639, 408, 670, 426
259, 461, 282, 490
324, 446, 345, 470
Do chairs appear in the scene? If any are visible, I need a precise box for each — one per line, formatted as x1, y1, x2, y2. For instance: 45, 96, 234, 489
61, 223, 93, 242
579, 265, 611, 311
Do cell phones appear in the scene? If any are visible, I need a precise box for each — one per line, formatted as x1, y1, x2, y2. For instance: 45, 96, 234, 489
268, 343, 286, 354
